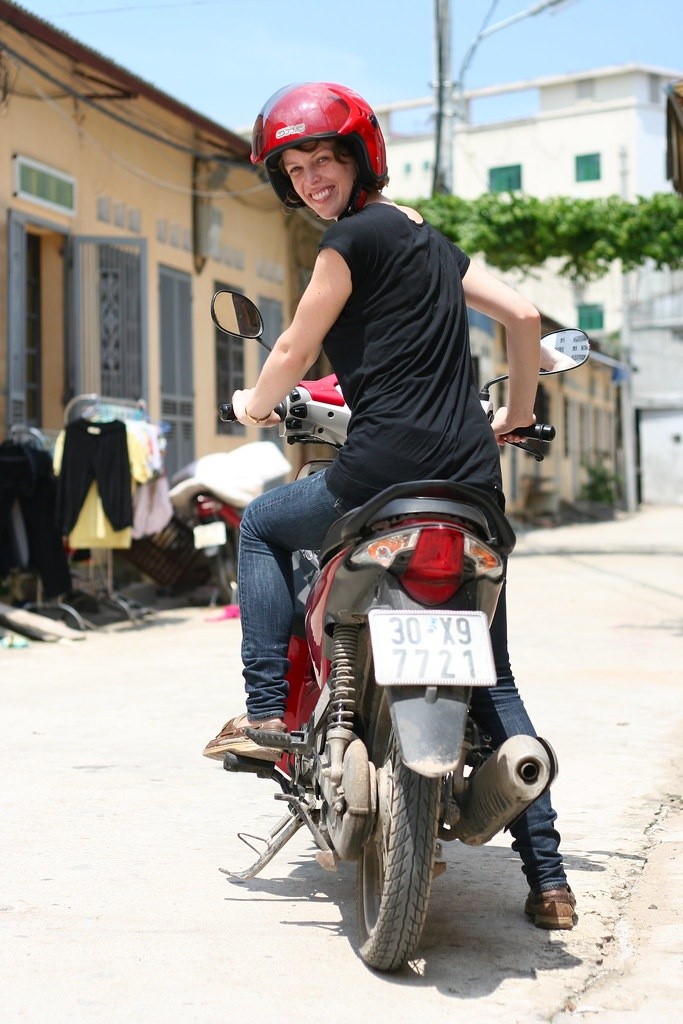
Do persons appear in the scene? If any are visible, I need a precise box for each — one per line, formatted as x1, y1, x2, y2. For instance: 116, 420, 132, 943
201, 81, 581, 933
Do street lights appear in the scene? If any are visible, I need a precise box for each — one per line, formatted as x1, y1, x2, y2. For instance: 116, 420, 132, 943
434, 0, 583, 195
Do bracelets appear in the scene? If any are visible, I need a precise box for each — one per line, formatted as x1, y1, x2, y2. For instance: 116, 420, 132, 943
244, 402, 273, 424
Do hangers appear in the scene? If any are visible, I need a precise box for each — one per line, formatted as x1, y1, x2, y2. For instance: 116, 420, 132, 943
0, 392, 171, 455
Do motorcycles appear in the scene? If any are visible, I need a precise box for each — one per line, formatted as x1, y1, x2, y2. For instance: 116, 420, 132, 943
198, 280, 592, 966
193, 489, 248, 607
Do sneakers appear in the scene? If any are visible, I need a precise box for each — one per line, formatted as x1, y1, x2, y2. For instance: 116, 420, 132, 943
203, 714, 283, 764
523, 889, 576, 927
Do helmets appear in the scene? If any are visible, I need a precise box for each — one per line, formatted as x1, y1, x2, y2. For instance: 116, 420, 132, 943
252, 83, 390, 208
